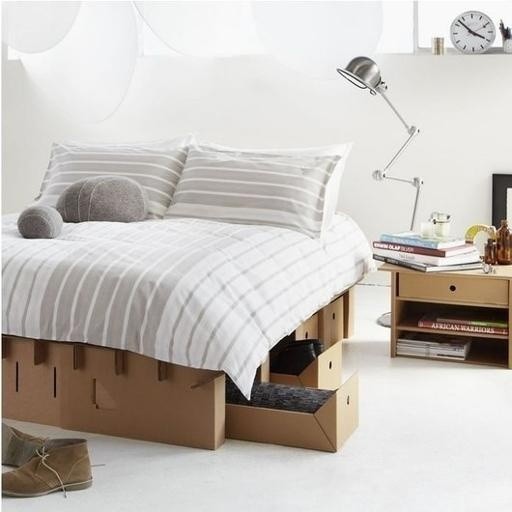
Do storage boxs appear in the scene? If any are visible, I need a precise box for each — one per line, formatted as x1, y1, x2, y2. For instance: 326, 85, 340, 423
225, 284, 361, 453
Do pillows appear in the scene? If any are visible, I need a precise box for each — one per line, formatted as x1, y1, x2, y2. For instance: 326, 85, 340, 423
56, 175, 147, 223
30, 133, 193, 222
164, 143, 348, 238
17, 203, 64, 237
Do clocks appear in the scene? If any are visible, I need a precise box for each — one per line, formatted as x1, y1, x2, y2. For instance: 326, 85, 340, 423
450, 11, 496, 55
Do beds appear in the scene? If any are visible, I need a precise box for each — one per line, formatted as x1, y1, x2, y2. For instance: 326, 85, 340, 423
1, 141, 378, 454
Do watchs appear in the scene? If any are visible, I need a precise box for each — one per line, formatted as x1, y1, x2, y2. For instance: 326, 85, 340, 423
483, 264, 498, 275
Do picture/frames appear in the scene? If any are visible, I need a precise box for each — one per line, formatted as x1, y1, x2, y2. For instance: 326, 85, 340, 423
491, 173, 512, 231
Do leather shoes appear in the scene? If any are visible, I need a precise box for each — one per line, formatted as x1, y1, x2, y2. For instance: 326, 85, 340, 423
270, 342, 323, 375
1, 437, 98, 499
1, 422, 49, 468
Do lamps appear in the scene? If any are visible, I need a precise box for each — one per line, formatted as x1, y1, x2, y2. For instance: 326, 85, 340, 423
335, 57, 427, 231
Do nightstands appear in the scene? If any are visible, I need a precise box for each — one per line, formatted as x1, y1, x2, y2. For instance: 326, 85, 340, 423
374, 250, 512, 370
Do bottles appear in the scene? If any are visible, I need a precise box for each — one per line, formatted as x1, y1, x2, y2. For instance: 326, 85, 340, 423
484, 219, 512, 265
432, 38, 443, 55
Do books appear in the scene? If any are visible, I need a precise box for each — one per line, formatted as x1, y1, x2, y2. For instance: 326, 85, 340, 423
396, 331, 471, 360
416, 310, 508, 336
372, 230, 487, 273
436, 308, 508, 330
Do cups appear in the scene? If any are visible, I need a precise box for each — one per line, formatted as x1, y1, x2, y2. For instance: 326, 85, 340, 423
422, 221, 434, 238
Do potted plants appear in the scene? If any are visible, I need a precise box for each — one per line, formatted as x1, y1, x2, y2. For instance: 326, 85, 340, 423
499, 19, 512, 54
